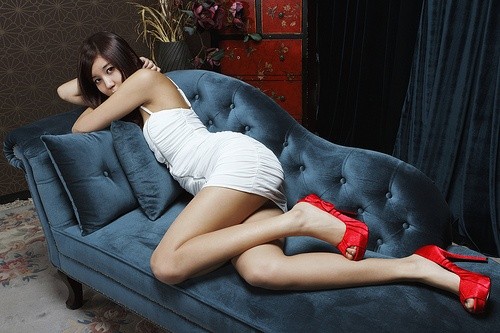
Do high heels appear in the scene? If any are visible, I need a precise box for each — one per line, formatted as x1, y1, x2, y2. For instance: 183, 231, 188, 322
414, 243, 490, 316
293, 193, 368, 260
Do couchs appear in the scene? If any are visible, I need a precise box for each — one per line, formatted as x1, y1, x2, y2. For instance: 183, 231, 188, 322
5, 70, 500, 333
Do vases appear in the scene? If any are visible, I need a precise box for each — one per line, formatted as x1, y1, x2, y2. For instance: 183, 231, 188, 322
126, 0, 262, 75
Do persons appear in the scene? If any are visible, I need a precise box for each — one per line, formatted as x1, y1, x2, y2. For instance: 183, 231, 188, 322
57, 32, 491, 315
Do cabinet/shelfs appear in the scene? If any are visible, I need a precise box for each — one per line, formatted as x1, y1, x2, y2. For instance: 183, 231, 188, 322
209, 0, 316, 136
